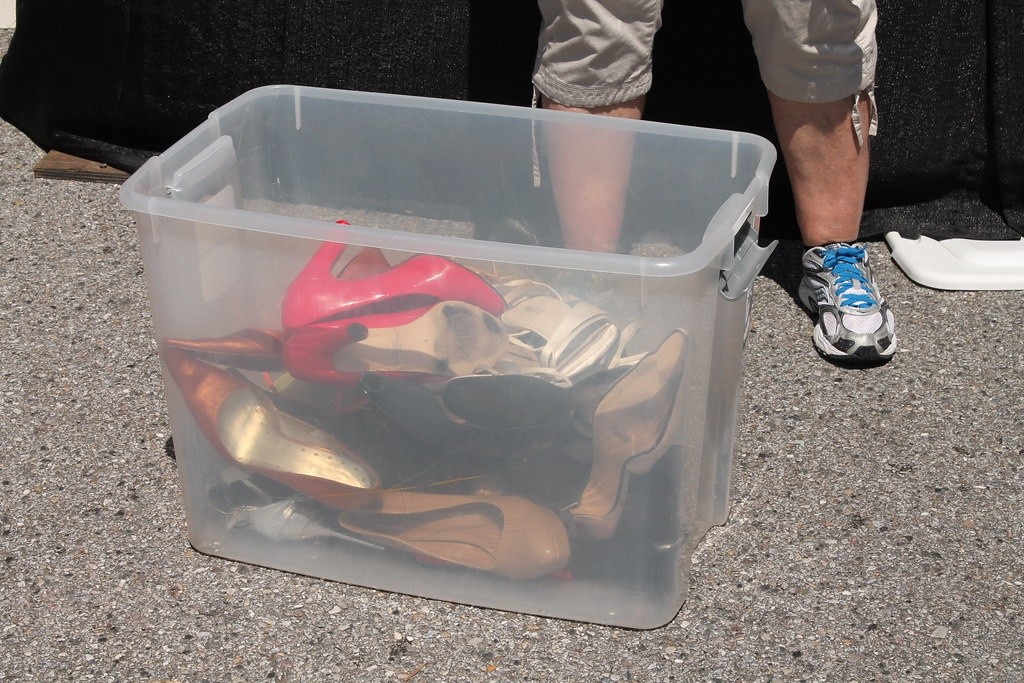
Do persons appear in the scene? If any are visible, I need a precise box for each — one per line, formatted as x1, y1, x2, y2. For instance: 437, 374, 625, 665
531, 0, 898, 364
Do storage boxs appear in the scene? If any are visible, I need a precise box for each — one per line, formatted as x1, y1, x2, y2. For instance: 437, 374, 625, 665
118, 85, 778, 630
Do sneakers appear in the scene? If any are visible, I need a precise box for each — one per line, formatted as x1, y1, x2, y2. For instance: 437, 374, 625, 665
797, 241, 897, 368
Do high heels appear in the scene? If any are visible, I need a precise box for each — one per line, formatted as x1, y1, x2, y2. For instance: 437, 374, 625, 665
162, 216, 686, 581
